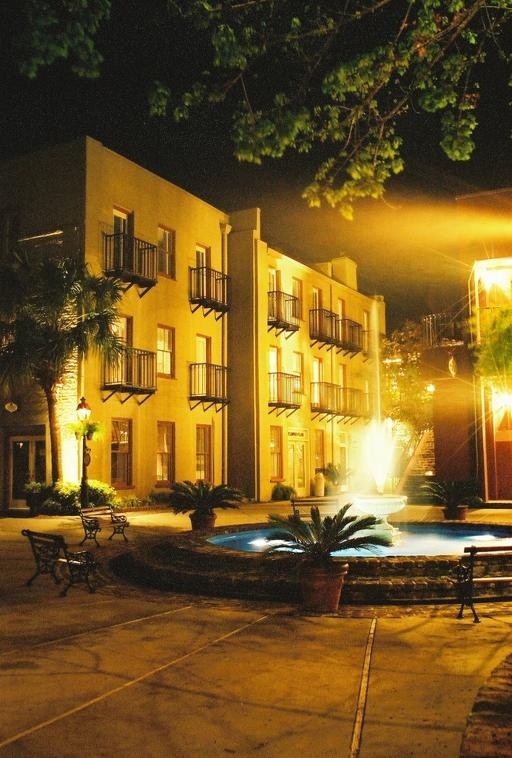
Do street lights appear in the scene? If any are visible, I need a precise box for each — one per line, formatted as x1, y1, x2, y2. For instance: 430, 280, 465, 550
75, 394, 94, 509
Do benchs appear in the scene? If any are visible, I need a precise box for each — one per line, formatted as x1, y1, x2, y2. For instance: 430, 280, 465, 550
290, 497, 341, 517
441, 544, 512, 624
21, 527, 97, 597
76, 503, 130, 547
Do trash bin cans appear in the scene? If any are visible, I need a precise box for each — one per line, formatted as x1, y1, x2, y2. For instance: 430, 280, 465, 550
314, 473, 324, 496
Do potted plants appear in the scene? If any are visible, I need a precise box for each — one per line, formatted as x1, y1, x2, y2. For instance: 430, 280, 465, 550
167, 477, 245, 530
262, 502, 396, 613
420, 478, 484, 519
